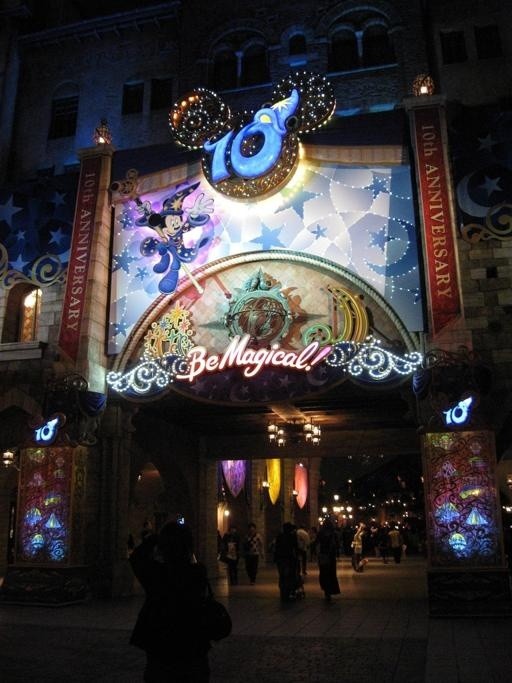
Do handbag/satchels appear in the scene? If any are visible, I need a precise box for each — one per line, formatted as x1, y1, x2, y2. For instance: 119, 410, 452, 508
198, 595, 232, 642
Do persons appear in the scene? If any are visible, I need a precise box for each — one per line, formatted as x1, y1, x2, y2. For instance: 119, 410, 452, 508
126, 518, 161, 557
126, 521, 231, 683
219, 514, 417, 604
